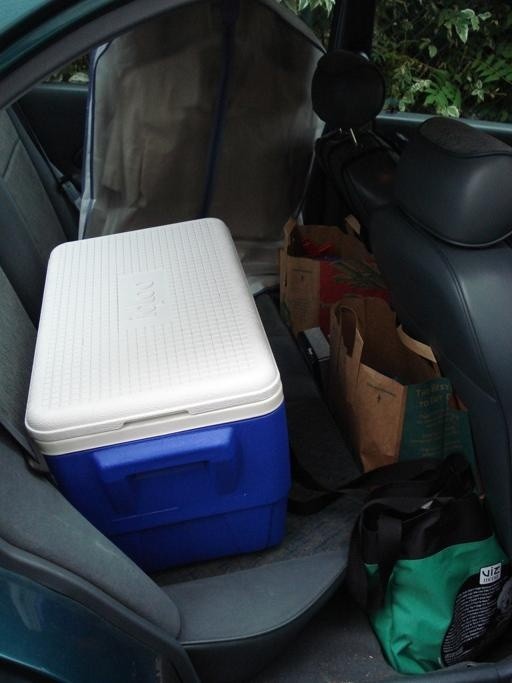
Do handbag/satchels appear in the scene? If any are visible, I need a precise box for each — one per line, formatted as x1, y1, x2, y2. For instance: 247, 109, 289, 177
277, 215, 390, 343
328, 295, 481, 502
334, 451, 512, 677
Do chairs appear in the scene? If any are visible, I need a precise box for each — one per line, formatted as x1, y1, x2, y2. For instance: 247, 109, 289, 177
0, 111, 372, 683
309, 48, 400, 247
373, 116, 511, 554
